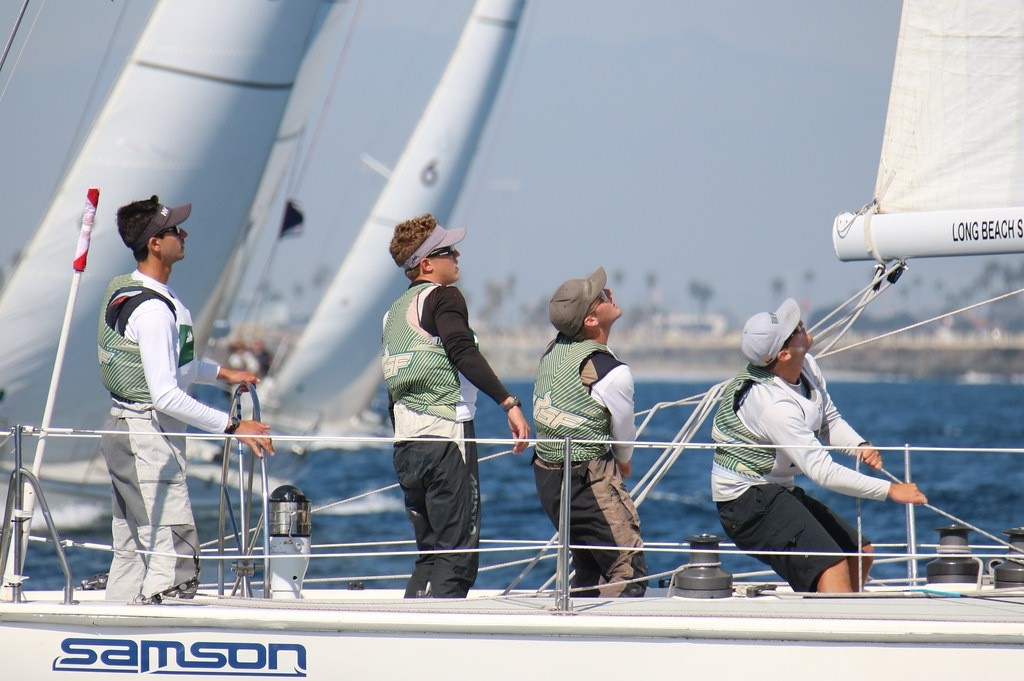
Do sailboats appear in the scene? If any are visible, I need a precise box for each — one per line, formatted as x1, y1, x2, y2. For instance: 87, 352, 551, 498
0, 0, 1024, 681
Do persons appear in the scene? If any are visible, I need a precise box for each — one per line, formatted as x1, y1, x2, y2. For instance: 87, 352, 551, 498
532, 267, 648, 598
381, 214, 530, 599
100, 196, 275, 603
711, 298, 927, 592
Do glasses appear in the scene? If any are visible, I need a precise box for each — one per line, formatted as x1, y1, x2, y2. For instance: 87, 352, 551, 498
784, 322, 803, 346
159, 224, 180, 236
588, 290, 607, 315
429, 244, 455, 257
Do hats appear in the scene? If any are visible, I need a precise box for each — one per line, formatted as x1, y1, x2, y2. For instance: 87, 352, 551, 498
130, 201, 191, 252
402, 223, 467, 270
741, 297, 800, 366
548, 266, 606, 338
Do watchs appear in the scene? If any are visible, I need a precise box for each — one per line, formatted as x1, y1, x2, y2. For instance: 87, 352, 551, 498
224, 416, 240, 434
504, 397, 521, 412
